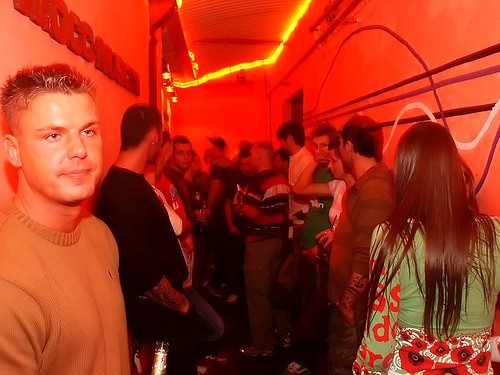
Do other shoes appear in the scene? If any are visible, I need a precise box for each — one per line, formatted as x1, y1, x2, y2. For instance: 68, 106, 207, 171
275, 329, 291, 347
283, 362, 308, 375
240, 344, 272, 357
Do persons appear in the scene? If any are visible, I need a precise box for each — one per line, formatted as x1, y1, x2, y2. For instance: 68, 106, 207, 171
94, 104, 396, 375
352, 121, 500, 375
0, 64, 133, 375
